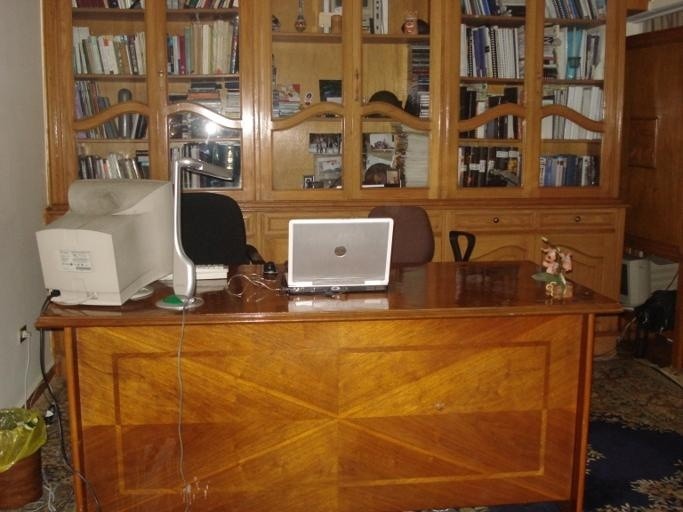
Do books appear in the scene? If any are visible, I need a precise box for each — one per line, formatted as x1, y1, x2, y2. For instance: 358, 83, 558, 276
269, 56, 302, 120
455, 2, 607, 190
404, 41, 430, 120
395, 133, 429, 189
362, 1, 389, 35
68, 1, 241, 189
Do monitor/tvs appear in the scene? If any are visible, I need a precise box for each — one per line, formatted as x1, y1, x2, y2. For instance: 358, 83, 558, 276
33, 177, 174, 307
619, 254, 679, 307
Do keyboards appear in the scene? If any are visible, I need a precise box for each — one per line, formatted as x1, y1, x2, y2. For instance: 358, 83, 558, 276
159, 264, 230, 281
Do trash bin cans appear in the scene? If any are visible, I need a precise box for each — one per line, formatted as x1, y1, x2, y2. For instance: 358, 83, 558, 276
0, 408, 47, 510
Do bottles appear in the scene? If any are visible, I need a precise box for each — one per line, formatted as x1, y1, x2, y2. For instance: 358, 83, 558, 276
295, 0, 306, 32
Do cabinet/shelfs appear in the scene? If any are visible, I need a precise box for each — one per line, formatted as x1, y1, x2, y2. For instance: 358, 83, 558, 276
440, 0, 625, 199
41, 0, 255, 202
444, 207, 627, 355
254, 0, 442, 204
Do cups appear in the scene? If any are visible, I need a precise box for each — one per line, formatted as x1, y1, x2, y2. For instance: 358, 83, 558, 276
118, 88, 133, 138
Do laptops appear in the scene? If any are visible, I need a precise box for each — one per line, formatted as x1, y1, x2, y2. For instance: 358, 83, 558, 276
282, 217, 394, 295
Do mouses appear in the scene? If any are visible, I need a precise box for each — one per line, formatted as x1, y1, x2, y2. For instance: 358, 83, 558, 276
264, 260, 279, 275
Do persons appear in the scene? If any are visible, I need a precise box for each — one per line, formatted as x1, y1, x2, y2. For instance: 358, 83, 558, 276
370, 139, 392, 149
313, 137, 338, 153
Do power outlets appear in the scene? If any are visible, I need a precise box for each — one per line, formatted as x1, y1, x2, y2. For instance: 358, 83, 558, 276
19, 324, 27, 344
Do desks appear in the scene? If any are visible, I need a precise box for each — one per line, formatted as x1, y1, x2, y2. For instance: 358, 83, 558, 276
35, 262, 625, 512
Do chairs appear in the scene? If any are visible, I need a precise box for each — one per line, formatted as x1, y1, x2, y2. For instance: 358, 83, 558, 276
368, 206, 434, 265
180, 193, 265, 267
